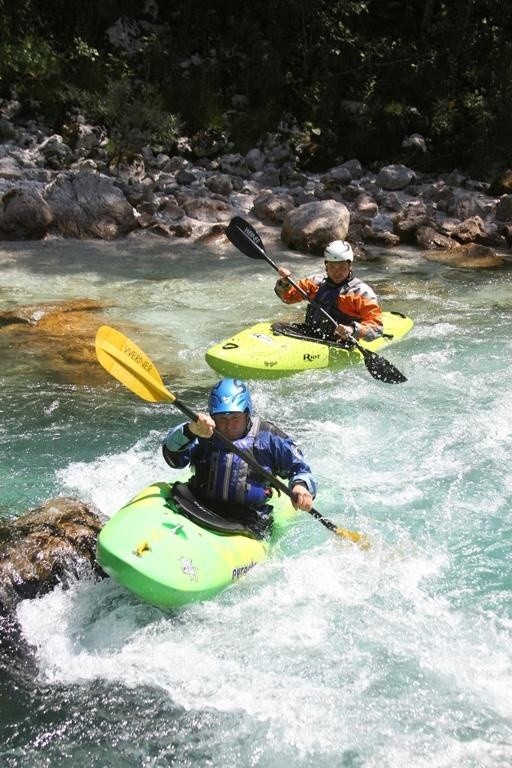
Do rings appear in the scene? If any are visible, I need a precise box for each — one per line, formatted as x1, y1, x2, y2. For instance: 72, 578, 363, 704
343, 330, 348, 334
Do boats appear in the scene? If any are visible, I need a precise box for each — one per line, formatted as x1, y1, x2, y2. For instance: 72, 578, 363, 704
95, 475, 302, 607
205, 310, 414, 382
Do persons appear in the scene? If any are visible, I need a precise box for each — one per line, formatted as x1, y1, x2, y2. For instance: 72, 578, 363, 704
160, 378, 317, 542
274, 238, 384, 350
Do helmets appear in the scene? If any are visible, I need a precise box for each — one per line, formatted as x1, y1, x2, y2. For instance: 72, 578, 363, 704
323, 238, 355, 264
207, 376, 252, 417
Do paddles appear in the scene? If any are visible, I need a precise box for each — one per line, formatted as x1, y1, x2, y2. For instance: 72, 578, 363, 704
225, 217, 407, 383
95, 325, 359, 543
